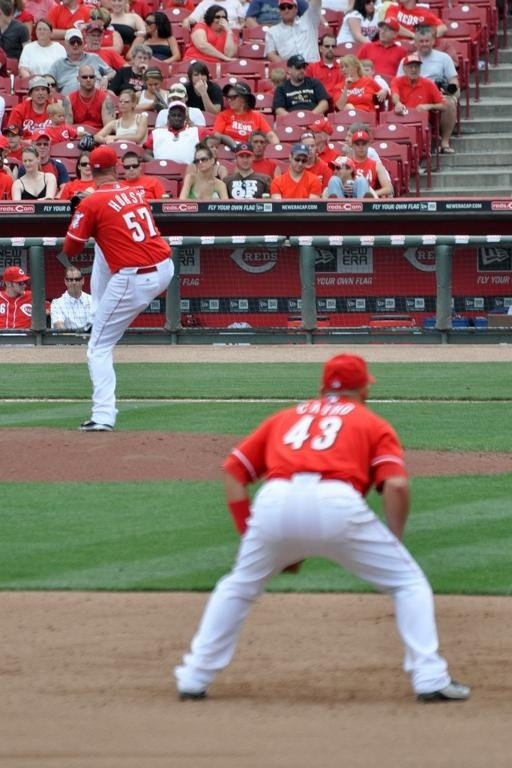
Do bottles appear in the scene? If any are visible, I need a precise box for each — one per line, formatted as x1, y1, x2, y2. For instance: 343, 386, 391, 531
211, 191, 219, 199
344, 179, 355, 198
261, 193, 270, 199
161, 193, 170, 199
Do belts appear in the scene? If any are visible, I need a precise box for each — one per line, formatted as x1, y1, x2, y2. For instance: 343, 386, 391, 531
136, 266, 157, 273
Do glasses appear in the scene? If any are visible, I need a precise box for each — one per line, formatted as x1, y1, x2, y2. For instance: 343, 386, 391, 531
145, 20, 157, 25
48, 39, 95, 87
193, 157, 211, 164
213, 16, 227, 19
80, 163, 91, 167
280, 5, 295, 9
65, 277, 82, 282
119, 99, 131, 104
124, 162, 141, 169
293, 64, 305, 70
238, 154, 251, 158
34, 142, 51, 147
323, 45, 337, 48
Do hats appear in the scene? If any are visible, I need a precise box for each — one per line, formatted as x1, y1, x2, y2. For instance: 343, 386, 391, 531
89, 146, 118, 169
32, 130, 50, 141
3, 267, 30, 282
27, 77, 50, 95
279, 0, 297, 6
2, 124, 23, 136
378, 18, 399, 32
167, 83, 256, 112
86, 23, 103, 33
235, 143, 253, 155
287, 55, 309, 67
325, 355, 376, 392
291, 119, 370, 175
404, 55, 423, 65
65, 29, 83, 40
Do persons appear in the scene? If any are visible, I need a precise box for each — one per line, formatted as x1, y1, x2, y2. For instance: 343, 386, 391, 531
1, 267, 51, 328
1, 1, 461, 201
50, 265, 94, 331
170, 353, 473, 703
65, 144, 174, 431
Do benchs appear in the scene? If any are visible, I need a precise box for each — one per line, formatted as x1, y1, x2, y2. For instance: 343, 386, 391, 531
0, 0, 509, 199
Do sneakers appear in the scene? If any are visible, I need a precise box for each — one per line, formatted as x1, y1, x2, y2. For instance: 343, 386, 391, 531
176, 689, 208, 701
79, 420, 114, 433
417, 681, 471, 703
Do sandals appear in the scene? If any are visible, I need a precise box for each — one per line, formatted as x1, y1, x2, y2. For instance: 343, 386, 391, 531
438, 144, 454, 153
